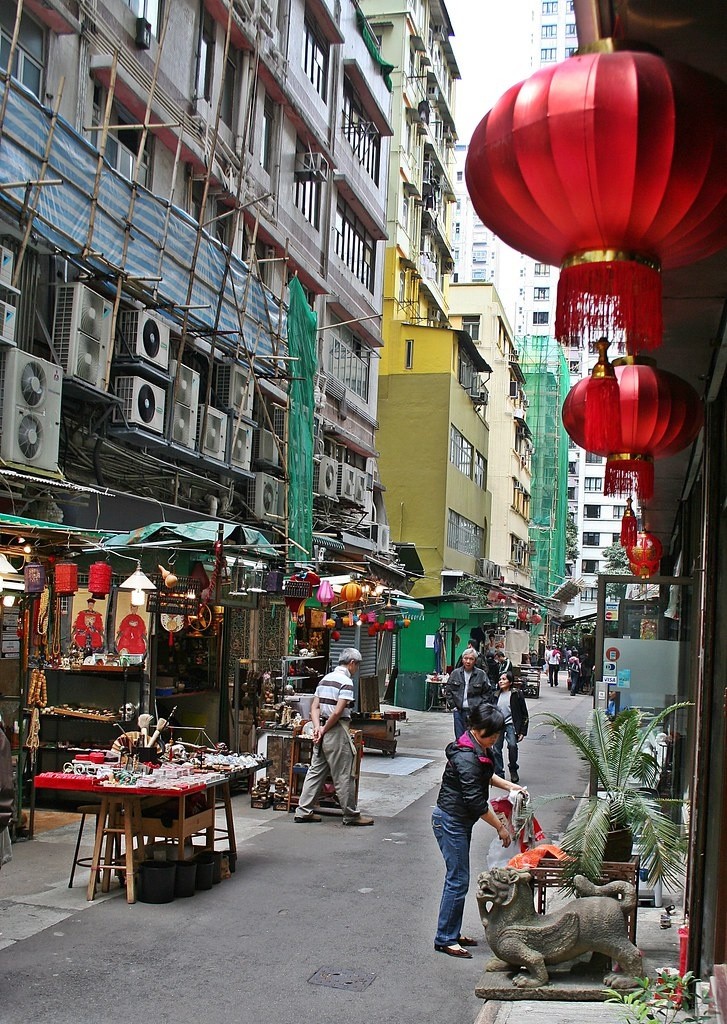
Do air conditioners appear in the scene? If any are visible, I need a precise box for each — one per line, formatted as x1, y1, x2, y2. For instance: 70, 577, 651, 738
369, 522, 389, 552
0, 246, 367, 522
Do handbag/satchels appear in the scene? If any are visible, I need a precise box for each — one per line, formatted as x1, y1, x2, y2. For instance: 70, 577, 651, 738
569, 661, 580, 672
543, 661, 549, 675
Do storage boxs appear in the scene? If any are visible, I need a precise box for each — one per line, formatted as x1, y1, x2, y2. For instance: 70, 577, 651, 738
119, 806, 214, 837
155, 685, 176, 697
119, 844, 210, 877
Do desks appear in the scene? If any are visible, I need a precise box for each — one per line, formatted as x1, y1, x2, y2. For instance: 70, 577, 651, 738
35, 756, 273, 905
428, 680, 448, 711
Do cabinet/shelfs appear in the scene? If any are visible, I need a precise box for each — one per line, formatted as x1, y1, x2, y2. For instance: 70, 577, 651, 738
287, 673, 408, 813
510, 665, 542, 698
27, 661, 144, 789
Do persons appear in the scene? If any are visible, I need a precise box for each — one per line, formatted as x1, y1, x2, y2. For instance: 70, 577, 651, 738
604, 691, 626, 715
294, 647, 374, 825
446, 639, 529, 782
432, 703, 526, 958
544, 645, 593, 697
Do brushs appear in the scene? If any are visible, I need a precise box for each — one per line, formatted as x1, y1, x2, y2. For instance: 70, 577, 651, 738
138, 714, 154, 747
146, 718, 170, 748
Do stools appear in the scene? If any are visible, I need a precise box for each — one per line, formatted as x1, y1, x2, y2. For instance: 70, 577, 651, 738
67, 805, 125, 889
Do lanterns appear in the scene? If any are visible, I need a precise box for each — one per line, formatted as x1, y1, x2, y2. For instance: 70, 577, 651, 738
519, 610, 530, 620
23, 563, 43, 596
341, 583, 363, 626
531, 613, 542, 625
55, 560, 78, 597
464, 36, 727, 450
562, 354, 702, 549
317, 580, 335, 624
265, 568, 284, 593
88, 561, 112, 599
625, 532, 663, 577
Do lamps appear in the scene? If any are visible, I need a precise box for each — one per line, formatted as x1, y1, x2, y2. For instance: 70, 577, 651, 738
107, 549, 157, 606
8, 534, 26, 546
228, 552, 270, 595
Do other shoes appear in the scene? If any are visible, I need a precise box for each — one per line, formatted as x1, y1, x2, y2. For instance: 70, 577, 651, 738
570, 690, 574, 696
295, 814, 322, 822
435, 942, 472, 959
458, 935, 477, 947
509, 770, 519, 782
344, 815, 374, 825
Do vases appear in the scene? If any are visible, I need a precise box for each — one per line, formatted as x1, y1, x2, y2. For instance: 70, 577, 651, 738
138, 851, 237, 903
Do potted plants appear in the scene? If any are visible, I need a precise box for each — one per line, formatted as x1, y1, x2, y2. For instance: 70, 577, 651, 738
513, 700, 695, 902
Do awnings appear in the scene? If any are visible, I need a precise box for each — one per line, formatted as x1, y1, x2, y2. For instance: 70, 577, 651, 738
0, 468, 117, 544
469, 575, 597, 629
385, 595, 425, 621
